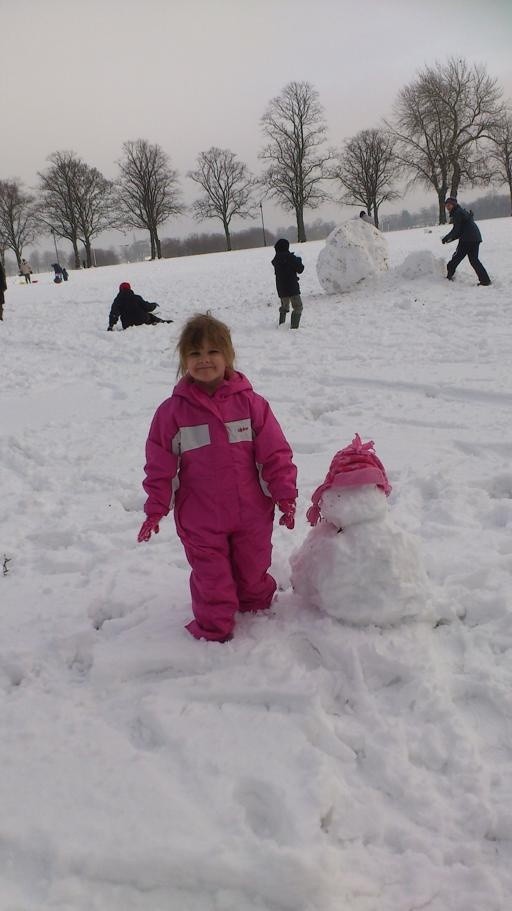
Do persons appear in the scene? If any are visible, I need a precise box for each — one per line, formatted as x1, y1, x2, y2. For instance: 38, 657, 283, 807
137, 311, 297, 648
441, 197, 491, 287
62, 267, 69, 281
271, 238, 304, 329
21, 259, 33, 284
359, 211, 374, 226
0, 261, 8, 321
52, 263, 63, 283
107, 282, 174, 331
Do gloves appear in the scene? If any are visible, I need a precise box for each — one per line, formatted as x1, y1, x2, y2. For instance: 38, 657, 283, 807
277, 498, 298, 529
136, 513, 160, 542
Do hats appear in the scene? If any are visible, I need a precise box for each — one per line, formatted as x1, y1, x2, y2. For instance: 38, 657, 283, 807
326, 448, 392, 497
445, 197, 457, 206
119, 282, 130, 291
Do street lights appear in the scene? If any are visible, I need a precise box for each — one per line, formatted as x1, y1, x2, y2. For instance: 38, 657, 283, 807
260, 203, 266, 246
50, 228, 60, 265
